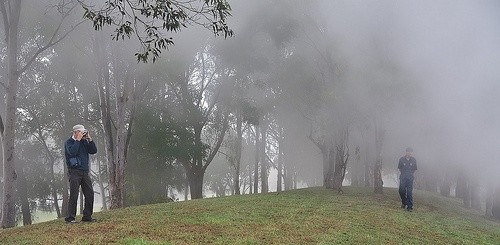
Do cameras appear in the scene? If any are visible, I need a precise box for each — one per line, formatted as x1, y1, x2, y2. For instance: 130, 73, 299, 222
83, 132, 88, 137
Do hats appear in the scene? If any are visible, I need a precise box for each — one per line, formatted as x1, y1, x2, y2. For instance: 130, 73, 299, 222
72, 124, 85, 132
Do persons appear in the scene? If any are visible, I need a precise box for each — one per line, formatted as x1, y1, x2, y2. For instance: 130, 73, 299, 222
65, 124, 97, 224
398, 148, 417, 209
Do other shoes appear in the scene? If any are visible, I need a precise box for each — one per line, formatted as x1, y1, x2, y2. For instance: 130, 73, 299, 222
64, 218, 81, 224
82, 217, 98, 222
400, 204, 414, 210
